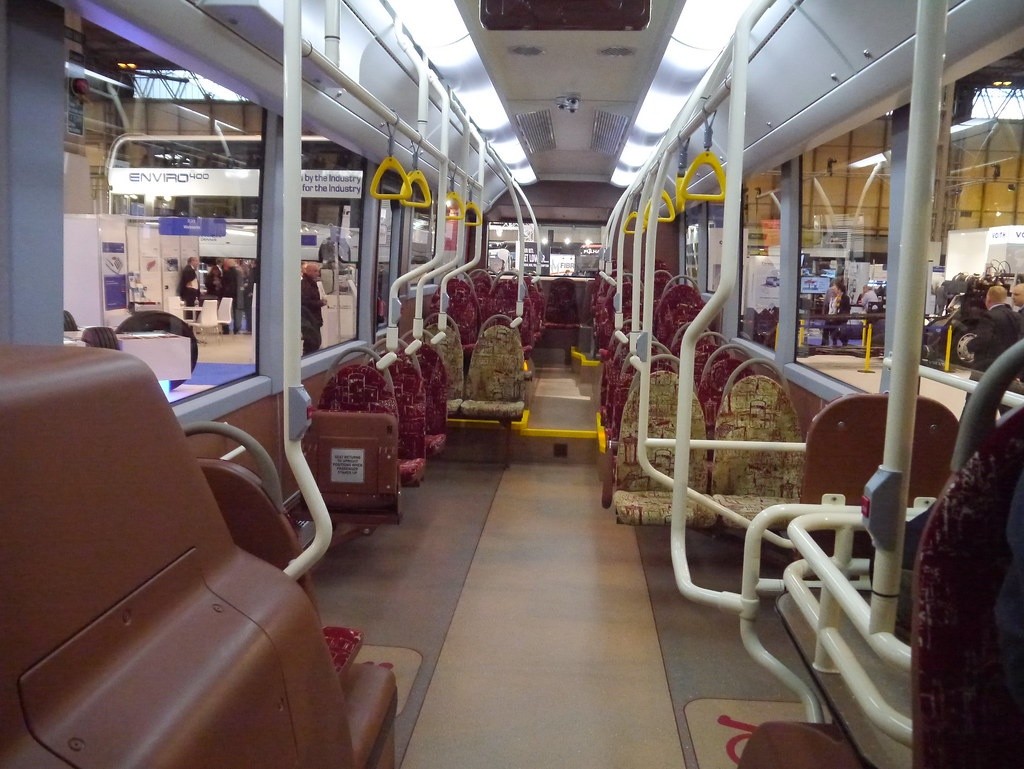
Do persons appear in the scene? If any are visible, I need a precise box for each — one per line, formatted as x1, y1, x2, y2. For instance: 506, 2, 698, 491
821, 279, 852, 345
301, 263, 328, 357
183, 257, 204, 327
936, 282, 954, 316
204, 257, 257, 334
966, 286, 1024, 416
1011, 283, 1024, 315
856, 285, 878, 312
563, 269, 578, 275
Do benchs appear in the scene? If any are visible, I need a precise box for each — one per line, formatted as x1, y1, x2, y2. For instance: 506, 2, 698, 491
582, 259, 803, 532
421, 269, 546, 470
541, 278, 580, 329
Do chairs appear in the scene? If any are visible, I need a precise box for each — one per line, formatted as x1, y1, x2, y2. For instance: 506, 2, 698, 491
800, 394, 959, 580
168, 295, 233, 347
308, 328, 450, 526
912, 340, 1024, 768
179, 421, 365, 685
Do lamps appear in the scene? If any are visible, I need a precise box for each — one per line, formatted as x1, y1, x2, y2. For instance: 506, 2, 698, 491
609, 0, 751, 188
383, 0, 540, 186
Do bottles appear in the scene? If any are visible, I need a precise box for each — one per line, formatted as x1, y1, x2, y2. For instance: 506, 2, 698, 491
194, 296, 199, 307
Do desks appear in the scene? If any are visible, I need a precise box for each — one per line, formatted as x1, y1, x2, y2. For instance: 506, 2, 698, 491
176, 307, 206, 344
